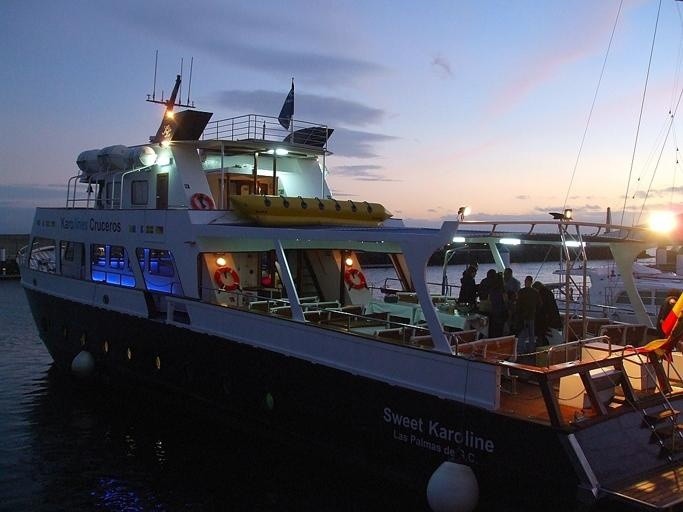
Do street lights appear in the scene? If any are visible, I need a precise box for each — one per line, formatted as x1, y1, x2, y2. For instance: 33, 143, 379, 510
13, 0, 682, 512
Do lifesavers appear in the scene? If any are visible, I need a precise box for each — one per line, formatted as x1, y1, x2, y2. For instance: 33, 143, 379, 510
345, 268, 366, 289
215, 267, 240, 291
191, 193, 214, 210
657, 296, 682, 339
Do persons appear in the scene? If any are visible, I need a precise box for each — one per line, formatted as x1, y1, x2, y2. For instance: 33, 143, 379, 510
656, 289, 683, 352
455, 265, 563, 357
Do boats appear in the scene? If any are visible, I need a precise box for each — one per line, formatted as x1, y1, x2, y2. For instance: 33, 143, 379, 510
550, 214, 683, 328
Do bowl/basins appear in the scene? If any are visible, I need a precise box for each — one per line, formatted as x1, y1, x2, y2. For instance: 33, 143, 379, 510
435, 302, 475, 316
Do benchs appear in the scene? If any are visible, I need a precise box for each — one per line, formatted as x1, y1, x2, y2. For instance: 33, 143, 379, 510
246, 290, 644, 399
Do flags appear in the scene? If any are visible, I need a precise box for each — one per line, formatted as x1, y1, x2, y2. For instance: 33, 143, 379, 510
277, 77, 294, 130
